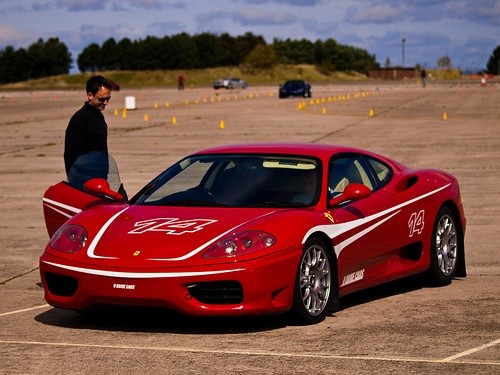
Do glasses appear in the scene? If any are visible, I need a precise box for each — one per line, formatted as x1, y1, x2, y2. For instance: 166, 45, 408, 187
93, 93, 111, 102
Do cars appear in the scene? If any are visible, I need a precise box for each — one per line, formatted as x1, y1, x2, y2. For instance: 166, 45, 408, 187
279, 78, 311, 100
213, 77, 248, 90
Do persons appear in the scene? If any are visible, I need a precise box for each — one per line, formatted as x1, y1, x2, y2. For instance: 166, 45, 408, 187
178, 74, 185, 90
421, 70, 426, 88
64, 75, 113, 198
291, 169, 341, 206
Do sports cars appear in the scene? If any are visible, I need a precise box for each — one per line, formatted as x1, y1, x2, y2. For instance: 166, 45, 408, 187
37, 142, 469, 327
